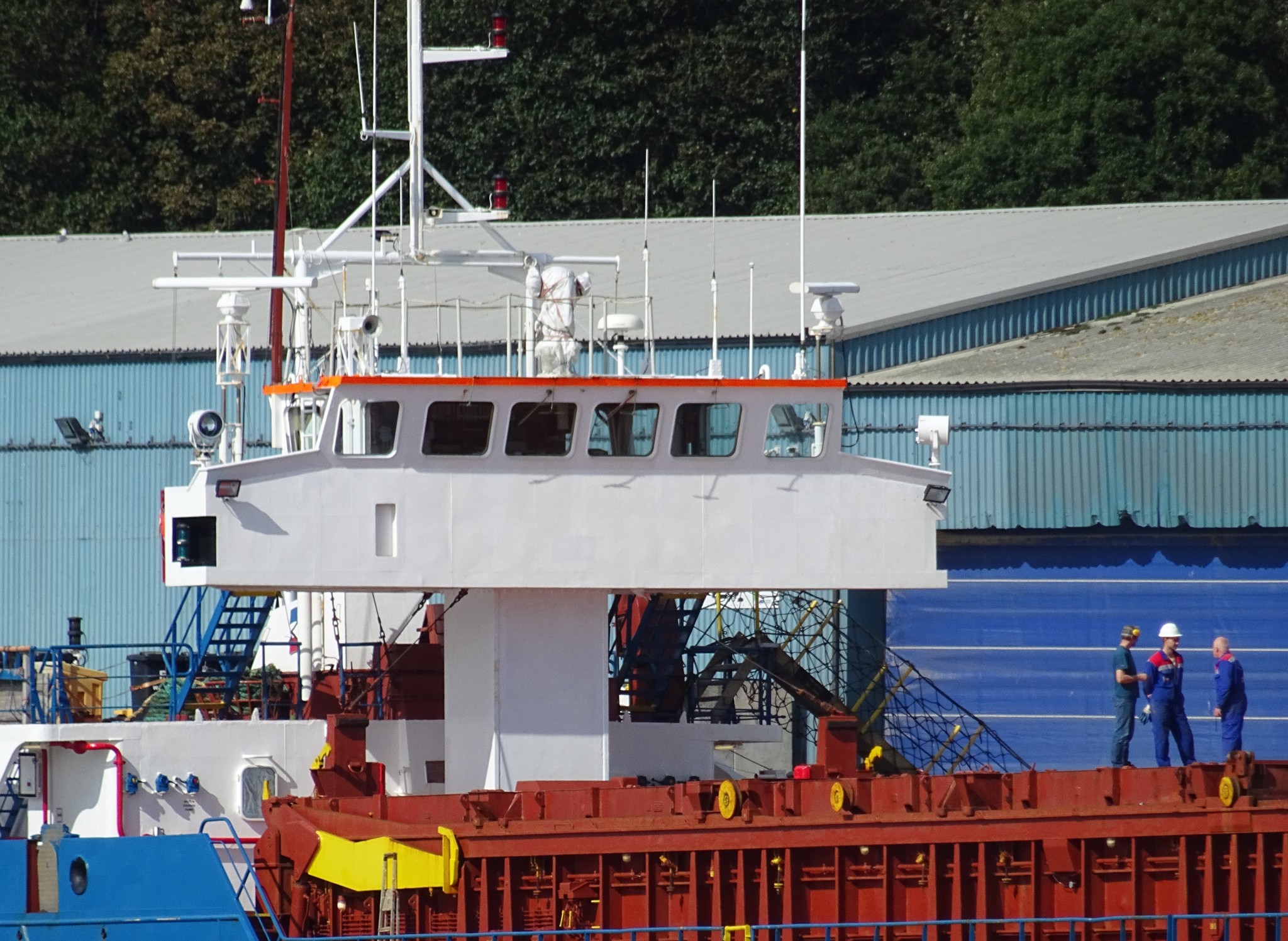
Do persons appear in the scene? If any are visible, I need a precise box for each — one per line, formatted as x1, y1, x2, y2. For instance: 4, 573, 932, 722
1111, 625, 1147, 768
1142, 622, 1196, 768
1212, 636, 1248, 764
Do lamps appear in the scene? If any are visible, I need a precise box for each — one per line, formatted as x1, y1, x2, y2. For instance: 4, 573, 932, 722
187, 410, 225, 466
923, 484, 951, 504
215, 479, 241, 498
54, 417, 91, 445
915, 415, 951, 469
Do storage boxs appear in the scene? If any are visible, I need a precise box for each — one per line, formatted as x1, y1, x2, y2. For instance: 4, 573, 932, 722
33, 660, 108, 723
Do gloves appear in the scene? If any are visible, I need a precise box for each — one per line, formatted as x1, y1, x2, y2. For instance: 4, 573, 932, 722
1139, 704, 1152, 725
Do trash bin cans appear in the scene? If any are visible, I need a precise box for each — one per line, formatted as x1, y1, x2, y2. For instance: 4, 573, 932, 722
184, 647, 243, 671
125, 650, 189, 722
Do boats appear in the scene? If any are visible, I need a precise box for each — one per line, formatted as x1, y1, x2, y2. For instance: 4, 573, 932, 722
0, 0, 1288, 941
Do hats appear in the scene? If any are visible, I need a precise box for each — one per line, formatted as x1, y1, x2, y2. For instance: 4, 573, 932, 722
1158, 623, 1183, 637
1121, 625, 1140, 639
